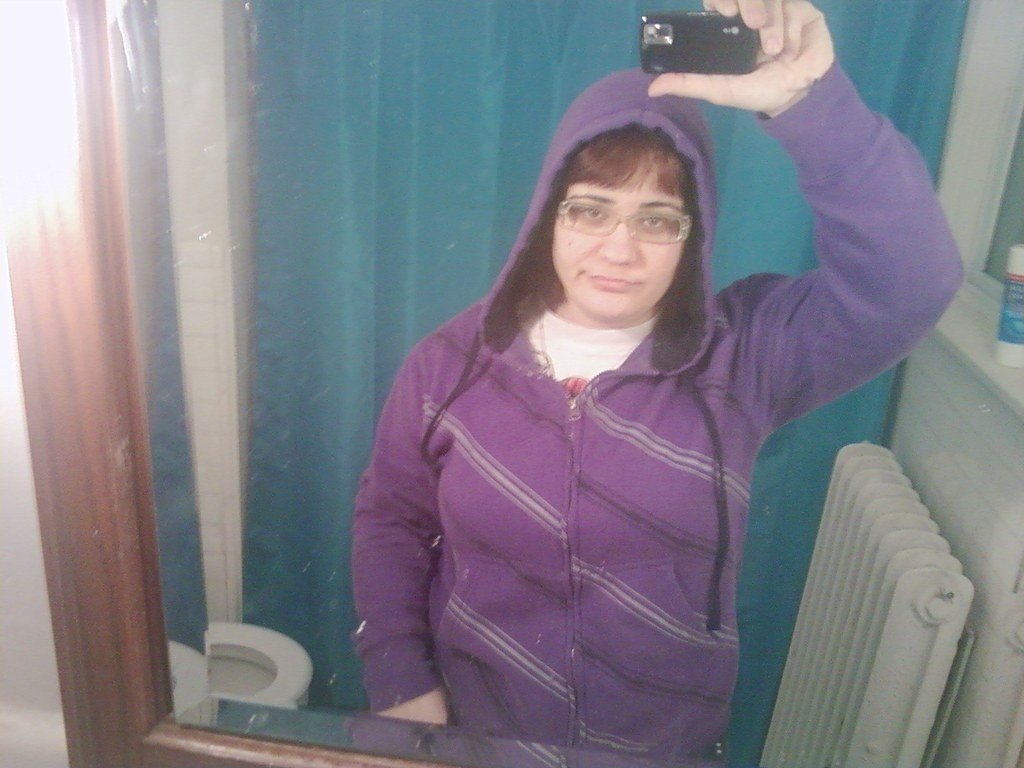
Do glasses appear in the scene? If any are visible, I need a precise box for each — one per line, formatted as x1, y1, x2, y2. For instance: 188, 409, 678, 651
556, 200, 691, 244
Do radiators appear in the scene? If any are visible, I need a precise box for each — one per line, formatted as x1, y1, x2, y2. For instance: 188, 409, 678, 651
760, 440, 974, 768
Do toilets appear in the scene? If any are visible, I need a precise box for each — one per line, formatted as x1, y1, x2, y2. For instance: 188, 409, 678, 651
209, 617, 315, 709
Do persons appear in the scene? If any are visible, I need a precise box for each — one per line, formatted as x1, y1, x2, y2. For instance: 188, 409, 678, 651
350, 0, 964, 762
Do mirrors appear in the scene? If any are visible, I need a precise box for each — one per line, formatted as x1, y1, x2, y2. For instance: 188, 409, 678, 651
0, 1, 1022, 768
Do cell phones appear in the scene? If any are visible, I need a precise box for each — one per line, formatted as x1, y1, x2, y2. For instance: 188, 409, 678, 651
640, 10, 760, 75
